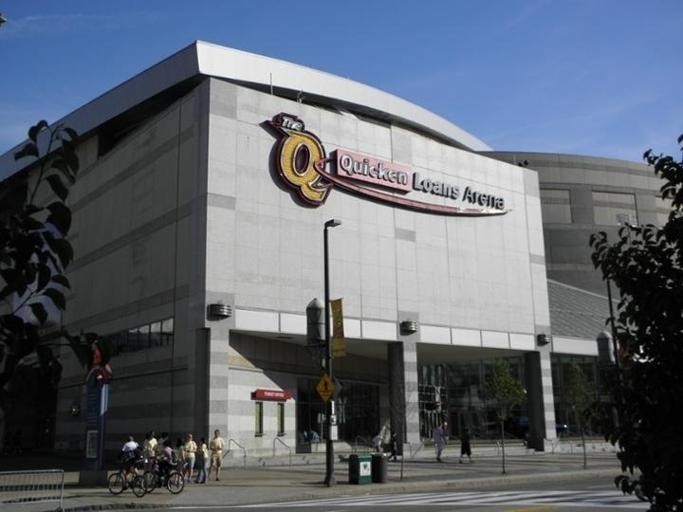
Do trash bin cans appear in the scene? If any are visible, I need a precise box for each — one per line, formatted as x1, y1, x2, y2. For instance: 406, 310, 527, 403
348, 454, 371, 484
372, 455, 386, 482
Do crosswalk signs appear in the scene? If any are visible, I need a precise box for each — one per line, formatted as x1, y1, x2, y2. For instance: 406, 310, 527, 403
316, 373, 336, 404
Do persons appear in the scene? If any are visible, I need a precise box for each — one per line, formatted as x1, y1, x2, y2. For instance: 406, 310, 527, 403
119, 426, 225, 488
460, 425, 475, 465
431, 422, 448, 462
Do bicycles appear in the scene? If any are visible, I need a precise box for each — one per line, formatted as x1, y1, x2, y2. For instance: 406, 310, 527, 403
108, 456, 184, 497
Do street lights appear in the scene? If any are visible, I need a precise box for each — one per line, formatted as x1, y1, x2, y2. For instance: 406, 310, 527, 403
324, 219, 341, 487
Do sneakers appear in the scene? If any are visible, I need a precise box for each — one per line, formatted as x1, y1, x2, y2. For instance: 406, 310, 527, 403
122, 477, 221, 490
